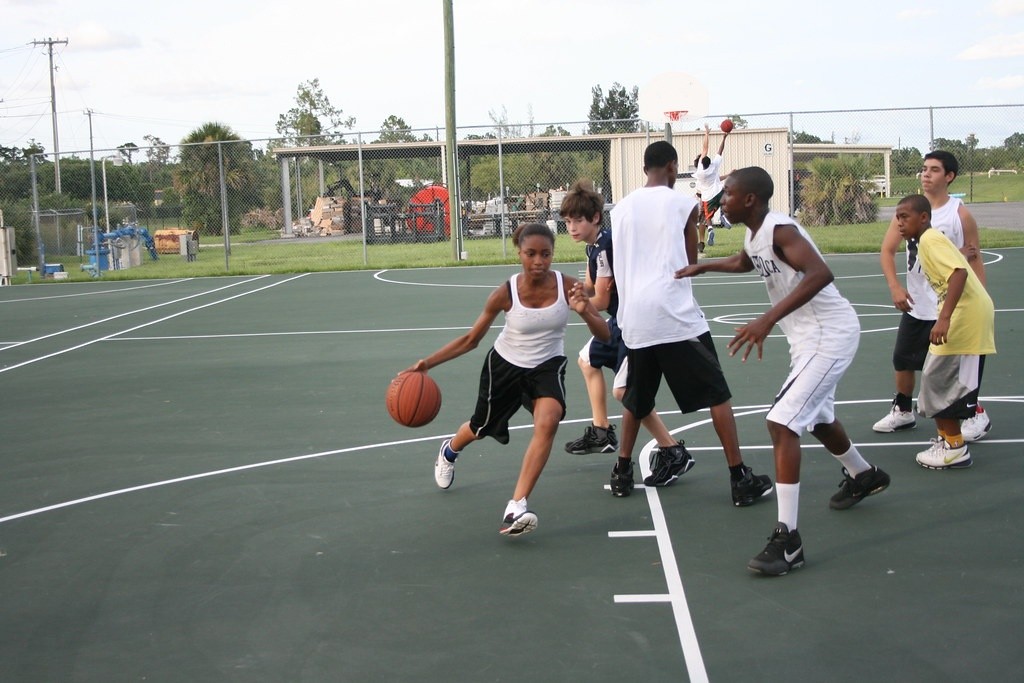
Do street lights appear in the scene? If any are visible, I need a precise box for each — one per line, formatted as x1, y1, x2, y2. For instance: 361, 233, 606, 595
101, 155, 116, 268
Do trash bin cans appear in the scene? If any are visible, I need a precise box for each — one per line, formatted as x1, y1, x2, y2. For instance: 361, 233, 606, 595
186, 232, 199, 261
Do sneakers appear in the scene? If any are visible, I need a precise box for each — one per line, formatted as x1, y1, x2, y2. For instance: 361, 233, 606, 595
610, 460, 634, 497
698, 242, 705, 253
961, 409, 992, 441
872, 405, 917, 432
565, 424, 617, 453
731, 467, 773, 506
831, 465, 890, 511
435, 439, 457, 489
720, 216, 731, 229
644, 440, 695, 485
915, 436, 972, 470
747, 522, 805, 578
707, 230, 714, 246
499, 497, 538, 537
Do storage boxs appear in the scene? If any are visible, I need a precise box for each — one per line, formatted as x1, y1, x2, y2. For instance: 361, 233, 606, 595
53, 272, 69, 280
311, 196, 373, 237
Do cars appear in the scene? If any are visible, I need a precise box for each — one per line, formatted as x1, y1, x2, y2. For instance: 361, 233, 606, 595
874, 176, 887, 192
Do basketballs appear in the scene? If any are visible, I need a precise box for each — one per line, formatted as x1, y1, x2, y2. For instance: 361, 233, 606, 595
720, 120, 733, 133
385, 370, 444, 429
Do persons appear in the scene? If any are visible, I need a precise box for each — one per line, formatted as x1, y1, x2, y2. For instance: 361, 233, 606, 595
895, 194, 997, 469
397, 222, 609, 537
872, 150, 993, 441
559, 190, 695, 487
674, 166, 891, 576
610, 140, 774, 506
693, 124, 736, 253
